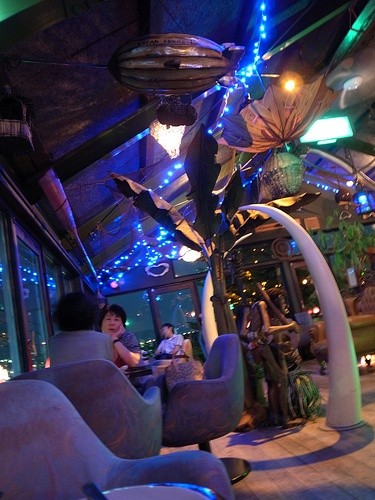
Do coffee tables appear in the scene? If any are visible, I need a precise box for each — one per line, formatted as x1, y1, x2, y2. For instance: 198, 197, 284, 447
81, 483, 221, 500
113, 366, 152, 379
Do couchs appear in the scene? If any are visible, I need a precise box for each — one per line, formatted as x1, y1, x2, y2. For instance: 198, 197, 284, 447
307, 280, 375, 376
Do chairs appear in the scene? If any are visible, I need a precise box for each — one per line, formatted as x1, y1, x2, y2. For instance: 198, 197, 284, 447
142, 334, 251, 489
8, 359, 161, 458
153, 339, 192, 376
0, 380, 232, 500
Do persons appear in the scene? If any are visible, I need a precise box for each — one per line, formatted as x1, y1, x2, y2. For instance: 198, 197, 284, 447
154, 322, 185, 358
95, 303, 146, 369
197, 314, 207, 359
48, 292, 118, 368
232, 287, 306, 431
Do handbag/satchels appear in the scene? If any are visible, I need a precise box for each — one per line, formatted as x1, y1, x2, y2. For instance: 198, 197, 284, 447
166, 349, 207, 392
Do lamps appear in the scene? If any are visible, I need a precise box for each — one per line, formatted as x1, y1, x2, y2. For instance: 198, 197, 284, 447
343, 147, 375, 225
